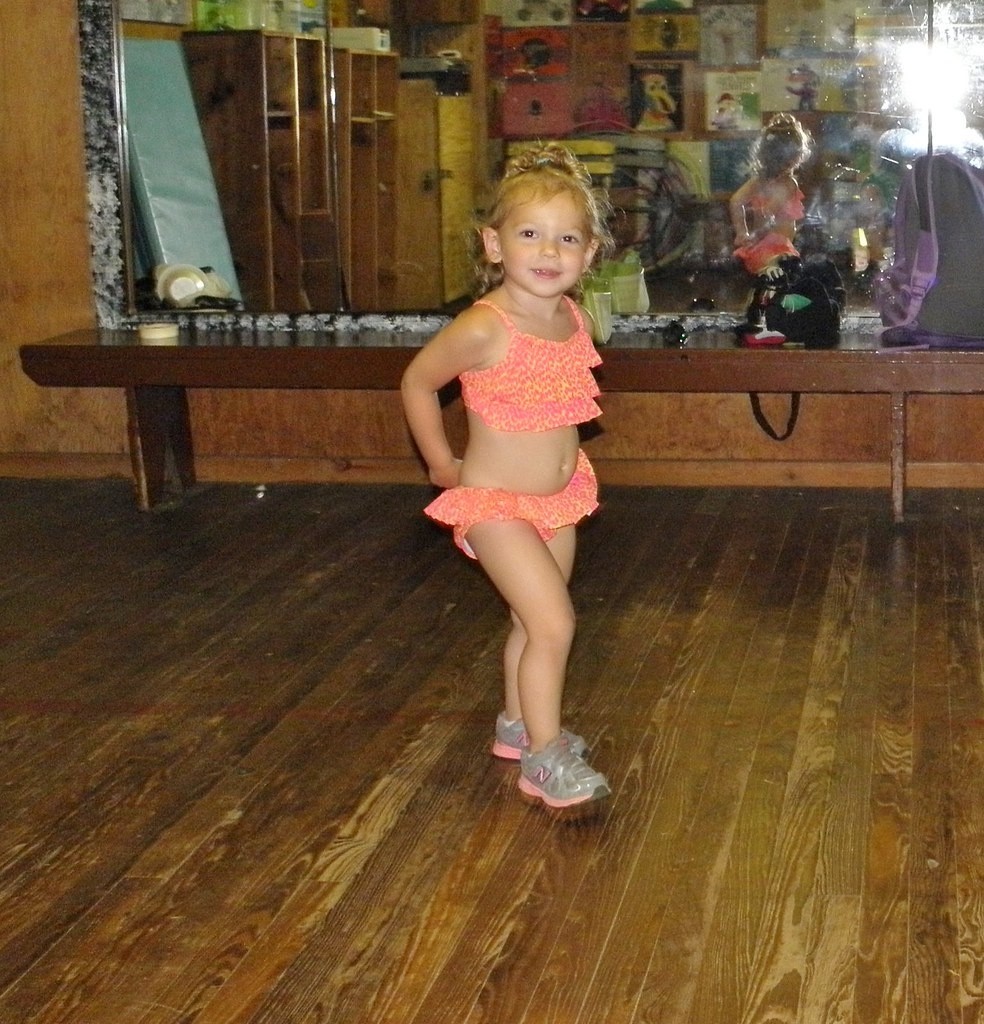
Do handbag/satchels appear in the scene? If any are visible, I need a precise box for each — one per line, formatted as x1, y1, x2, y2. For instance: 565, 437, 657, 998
746, 274, 841, 350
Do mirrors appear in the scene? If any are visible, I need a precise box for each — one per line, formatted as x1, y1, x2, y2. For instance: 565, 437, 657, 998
76, 0, 984, 355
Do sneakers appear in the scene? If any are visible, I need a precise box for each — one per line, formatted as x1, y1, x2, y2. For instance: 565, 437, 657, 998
516, 736, 614, 810
491, 709, 592, 762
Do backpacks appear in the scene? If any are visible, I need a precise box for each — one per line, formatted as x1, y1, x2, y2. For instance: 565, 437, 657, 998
871, 151, 983, 354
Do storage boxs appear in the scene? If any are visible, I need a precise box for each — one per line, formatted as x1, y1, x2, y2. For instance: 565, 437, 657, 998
312, 28, 390, 50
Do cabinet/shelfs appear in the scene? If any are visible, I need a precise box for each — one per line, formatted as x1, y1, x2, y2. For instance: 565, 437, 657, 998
180, 30, 473, 313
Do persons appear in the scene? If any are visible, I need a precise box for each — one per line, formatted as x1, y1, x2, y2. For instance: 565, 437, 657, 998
401, 140, 612, 807
859, 129, 919, 262
730, 112, 811, 273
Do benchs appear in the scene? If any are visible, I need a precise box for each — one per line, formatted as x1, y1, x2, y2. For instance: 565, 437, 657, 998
19, 326, 983, 522
